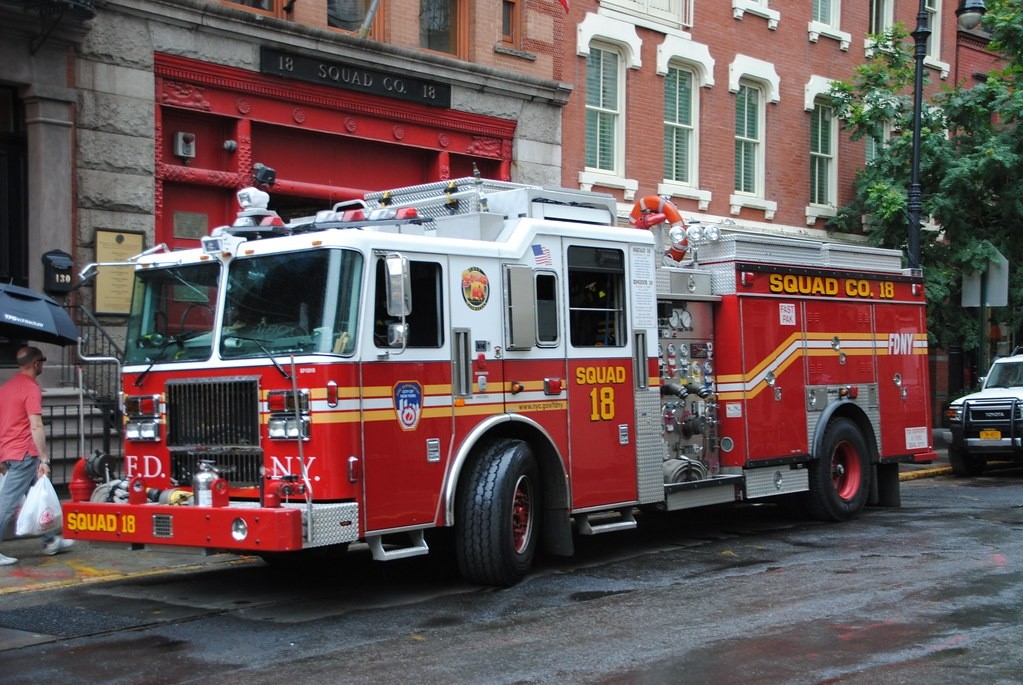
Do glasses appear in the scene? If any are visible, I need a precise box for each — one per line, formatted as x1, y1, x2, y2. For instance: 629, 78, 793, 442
34, 357, 47, 364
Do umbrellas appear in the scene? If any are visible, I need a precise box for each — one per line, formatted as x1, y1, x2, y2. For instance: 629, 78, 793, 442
0, 277, 86, 344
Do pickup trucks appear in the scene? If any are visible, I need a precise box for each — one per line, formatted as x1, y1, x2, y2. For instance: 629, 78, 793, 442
944, 345, 1023, 475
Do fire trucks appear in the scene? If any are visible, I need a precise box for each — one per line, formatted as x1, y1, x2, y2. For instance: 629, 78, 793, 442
59, 160, 935, 594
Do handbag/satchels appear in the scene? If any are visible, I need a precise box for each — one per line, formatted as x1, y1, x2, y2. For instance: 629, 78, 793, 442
16, 474, 63, 536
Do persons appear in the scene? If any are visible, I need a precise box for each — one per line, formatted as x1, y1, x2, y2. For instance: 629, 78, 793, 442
0, 347, 75, 565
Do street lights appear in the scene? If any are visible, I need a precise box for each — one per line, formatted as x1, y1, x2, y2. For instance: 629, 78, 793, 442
903, 1, 992, 269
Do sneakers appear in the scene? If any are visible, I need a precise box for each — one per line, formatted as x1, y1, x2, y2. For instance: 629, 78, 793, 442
40, 536, 74, 556
0, 554, 18, 565
